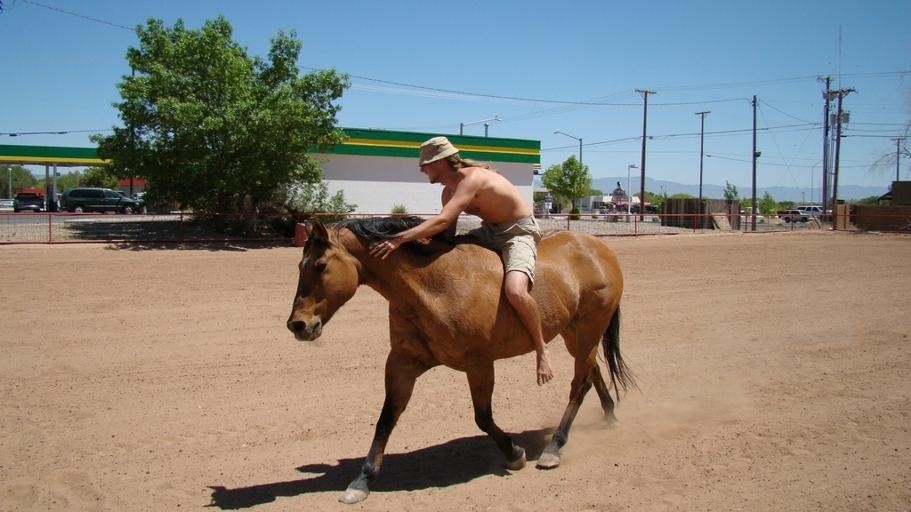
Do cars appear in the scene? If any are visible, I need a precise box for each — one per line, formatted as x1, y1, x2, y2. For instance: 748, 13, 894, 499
781, 206, 832, 224
13, 193, 40, 212
132, 192, 146, 207
745, 206, 759, 217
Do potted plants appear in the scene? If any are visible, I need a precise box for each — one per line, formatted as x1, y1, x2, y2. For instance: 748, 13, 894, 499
570, 207, 580, 220
391, 202, 407, 219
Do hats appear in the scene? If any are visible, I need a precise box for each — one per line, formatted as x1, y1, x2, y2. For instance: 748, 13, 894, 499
418, 136, 460, 166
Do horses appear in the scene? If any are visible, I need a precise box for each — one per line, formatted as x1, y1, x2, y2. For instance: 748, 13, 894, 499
285, 212, 637, 505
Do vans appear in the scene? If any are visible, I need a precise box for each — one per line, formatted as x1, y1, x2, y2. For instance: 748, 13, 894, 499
61, 187, 141, 215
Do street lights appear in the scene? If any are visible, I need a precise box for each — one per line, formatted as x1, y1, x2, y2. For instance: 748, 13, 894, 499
554, 130, 583, 216
460, 118, 503, 136
626, 165, 639, 224
8, 168, 12, 204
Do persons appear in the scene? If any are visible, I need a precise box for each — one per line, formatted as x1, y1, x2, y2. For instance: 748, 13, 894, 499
367, 136, 555, 385
